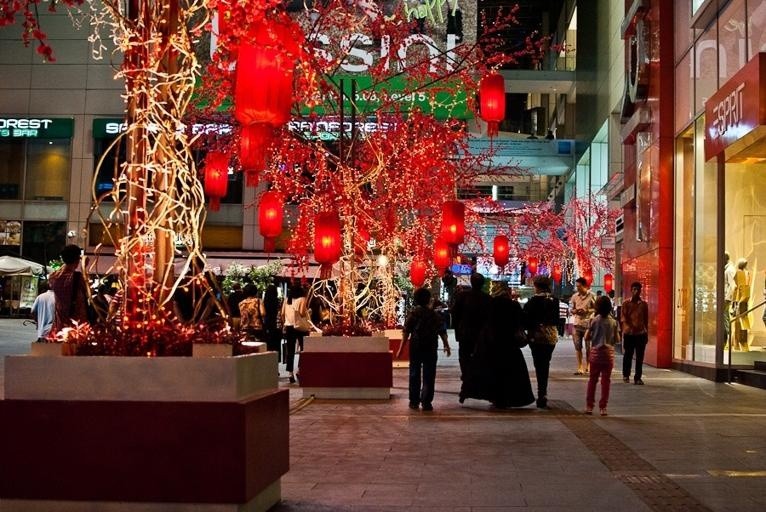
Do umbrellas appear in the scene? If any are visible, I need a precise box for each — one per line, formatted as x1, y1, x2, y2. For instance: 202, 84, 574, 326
0, 255, 45, 315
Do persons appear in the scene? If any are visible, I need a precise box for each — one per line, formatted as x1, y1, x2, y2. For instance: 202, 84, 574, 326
396, 270, 570, 414
731, 257, 754, 354
619, 282, 652, 385
567, 276, 597, 379
723, 251, 738, 350
30, 243, 327, 385
581, 297, 621, 420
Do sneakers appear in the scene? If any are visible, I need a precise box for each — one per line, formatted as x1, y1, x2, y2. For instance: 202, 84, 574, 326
585, 408, 607, 415
625, 376, 643, 383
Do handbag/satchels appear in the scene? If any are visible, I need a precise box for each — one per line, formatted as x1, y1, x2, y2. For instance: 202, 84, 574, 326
293, 310, 310, 332
283, 343, 288, 364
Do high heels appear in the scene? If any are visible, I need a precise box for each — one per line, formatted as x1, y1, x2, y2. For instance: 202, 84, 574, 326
289, 375, 296, 384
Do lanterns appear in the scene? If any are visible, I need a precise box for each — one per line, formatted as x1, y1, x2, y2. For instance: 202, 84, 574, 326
202, 40, 614, 294
